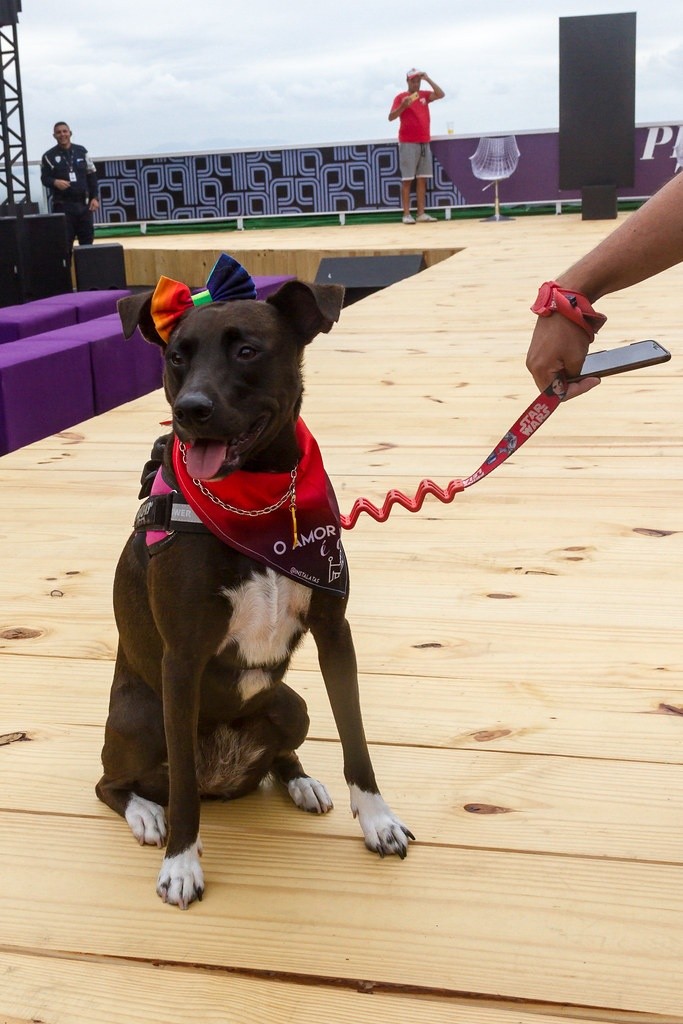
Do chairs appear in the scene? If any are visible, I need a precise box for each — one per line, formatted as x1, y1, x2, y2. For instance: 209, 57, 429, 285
468, 136, 521, 221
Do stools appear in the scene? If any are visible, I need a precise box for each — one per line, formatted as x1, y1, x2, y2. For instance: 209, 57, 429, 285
0, 274, 297, 457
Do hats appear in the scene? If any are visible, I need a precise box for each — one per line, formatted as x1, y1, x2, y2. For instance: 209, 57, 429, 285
407, 68, 422, 80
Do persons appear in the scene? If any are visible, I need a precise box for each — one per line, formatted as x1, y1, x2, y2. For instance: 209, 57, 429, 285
39, 120, 100, 247
524, 165, 683, 405
386, 67, 447, 225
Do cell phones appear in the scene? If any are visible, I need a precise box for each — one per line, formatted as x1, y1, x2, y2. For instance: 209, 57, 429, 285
565, 339, 671, 382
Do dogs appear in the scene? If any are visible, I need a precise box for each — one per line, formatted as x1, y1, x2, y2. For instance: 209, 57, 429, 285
95, 281, 417, 909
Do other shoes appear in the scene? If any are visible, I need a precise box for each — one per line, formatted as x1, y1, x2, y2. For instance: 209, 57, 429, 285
402, 215, 416, 224
416, 213, 437, 222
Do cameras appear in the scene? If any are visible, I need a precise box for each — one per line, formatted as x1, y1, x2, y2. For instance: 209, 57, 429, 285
410, 91, 420, 102
